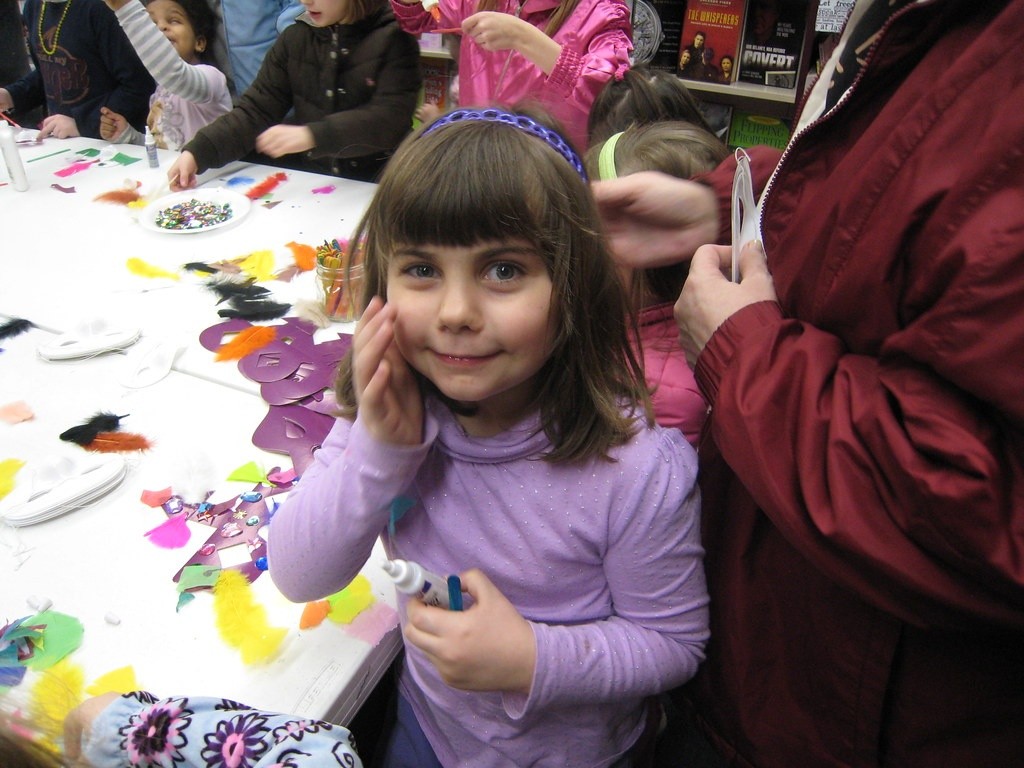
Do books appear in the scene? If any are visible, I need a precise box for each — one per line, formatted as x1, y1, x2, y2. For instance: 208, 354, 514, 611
648, 0, 809, 85
693, 99, 791, 154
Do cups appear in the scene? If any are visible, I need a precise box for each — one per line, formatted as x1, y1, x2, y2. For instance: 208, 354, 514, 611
313, 261, 365, 322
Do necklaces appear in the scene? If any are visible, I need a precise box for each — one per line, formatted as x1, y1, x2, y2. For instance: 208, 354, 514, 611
38, 0, 71, 55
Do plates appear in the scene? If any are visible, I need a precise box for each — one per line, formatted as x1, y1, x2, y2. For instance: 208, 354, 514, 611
139, 188, 251, 234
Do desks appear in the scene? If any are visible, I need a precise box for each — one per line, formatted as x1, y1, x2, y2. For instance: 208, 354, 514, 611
0, 126, 405, 768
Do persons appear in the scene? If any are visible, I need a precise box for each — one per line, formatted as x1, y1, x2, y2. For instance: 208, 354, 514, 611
413, 31, 462, 123
691, 47, 719, 82
717, 56, 732, 83
165, 0, 423, 192
582, 0, 1023, 768
205, 0, 307, 106
684, 31, 706, 65
98, 0, 235, 153
583, 63, 719, 144
0, 0, 157, 140
390, 1, 635, 157
578, 121, 730, 450
63, 691, 364, 768
677, 49, 691, 80
263, 98, 710, 768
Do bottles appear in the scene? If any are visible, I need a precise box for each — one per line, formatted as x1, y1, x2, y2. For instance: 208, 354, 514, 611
0, 119, 30, 192
380, 559, 449, 610
144, 126, 158, 167
